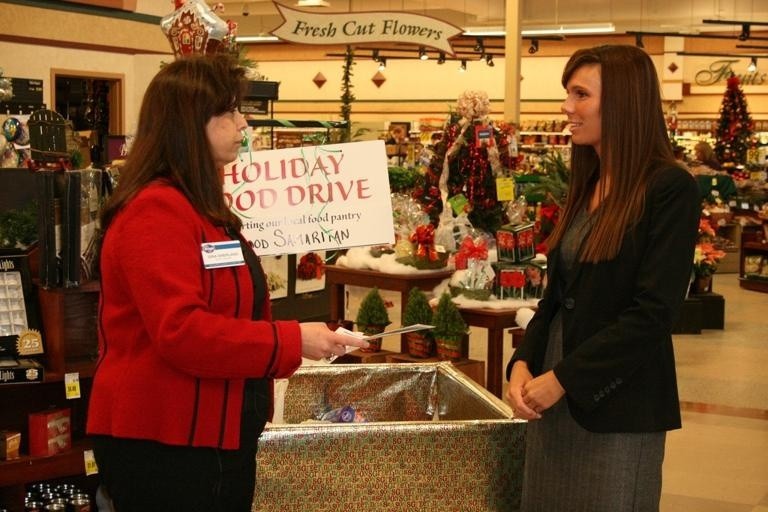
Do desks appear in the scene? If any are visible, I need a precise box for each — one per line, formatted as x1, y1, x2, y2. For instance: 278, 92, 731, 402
322, 260, 541, 400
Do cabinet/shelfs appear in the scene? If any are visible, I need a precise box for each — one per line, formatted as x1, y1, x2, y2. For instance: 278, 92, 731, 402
0, 234, 103, 511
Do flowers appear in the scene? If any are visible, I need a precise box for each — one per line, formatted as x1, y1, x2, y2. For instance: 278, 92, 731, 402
693, 243, 726, 276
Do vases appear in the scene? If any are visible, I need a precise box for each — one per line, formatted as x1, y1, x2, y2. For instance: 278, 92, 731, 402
693, 272, 711, 293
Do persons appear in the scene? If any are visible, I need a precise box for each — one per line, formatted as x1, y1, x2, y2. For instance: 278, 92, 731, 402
82, 57, 371, 511
383, 122, 414, 159
504, 45, 702, 511
694, 140, 723, 172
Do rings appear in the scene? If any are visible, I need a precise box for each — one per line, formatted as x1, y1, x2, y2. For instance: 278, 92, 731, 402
512, 408, 516, 413
